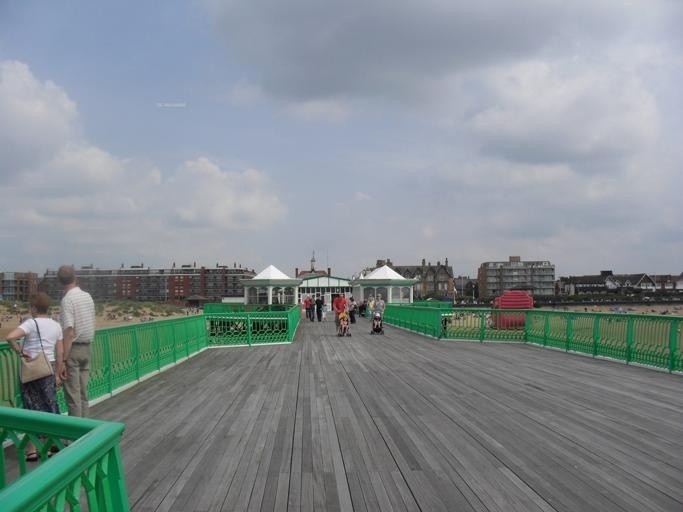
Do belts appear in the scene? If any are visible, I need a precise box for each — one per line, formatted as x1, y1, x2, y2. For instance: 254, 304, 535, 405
71, 341, 90, 345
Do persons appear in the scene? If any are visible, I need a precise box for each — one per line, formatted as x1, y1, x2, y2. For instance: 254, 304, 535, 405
303, 291, 385, 334
56, 266, 97, 448
5, 293, 64, 463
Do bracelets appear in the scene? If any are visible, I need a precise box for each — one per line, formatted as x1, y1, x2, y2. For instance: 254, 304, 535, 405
63, 359, 66, 363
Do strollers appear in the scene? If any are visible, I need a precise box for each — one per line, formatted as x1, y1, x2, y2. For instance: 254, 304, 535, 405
371, 311, 384, 335
338, 312, 351, 337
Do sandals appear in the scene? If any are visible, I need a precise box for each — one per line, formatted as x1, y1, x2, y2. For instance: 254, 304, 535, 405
24, 443, 70, 462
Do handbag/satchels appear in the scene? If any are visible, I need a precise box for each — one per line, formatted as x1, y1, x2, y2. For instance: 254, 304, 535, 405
16, 353, 53, 384
